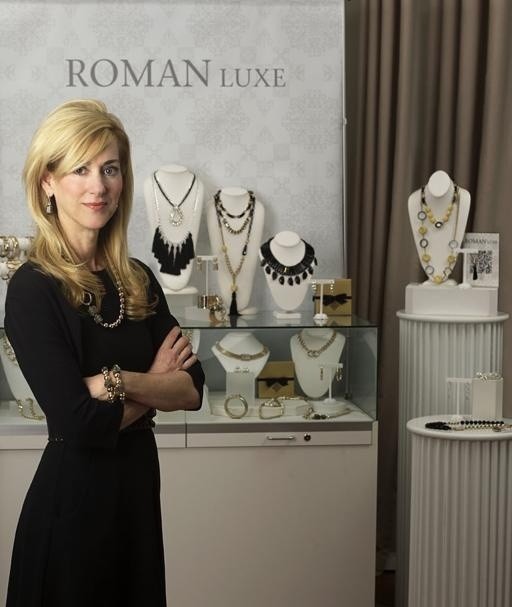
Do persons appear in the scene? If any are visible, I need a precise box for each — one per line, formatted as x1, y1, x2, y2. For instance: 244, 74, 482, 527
4, 101, 206, 607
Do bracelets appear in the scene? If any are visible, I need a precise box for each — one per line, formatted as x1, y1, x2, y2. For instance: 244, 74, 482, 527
0, 236, 37, 285
223, 394, 248, 419
102, 364, 125, 402
258, 395, 351, 420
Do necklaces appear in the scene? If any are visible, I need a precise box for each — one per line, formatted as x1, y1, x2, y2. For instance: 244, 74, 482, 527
298, 330, 337, 357
260, 237, 318, 284
16, 398, 44, 420
416, 184, 461, 283
88, 254, 125, 329
215, 341, 269, 361
425, 421, 510, 430
3, 336, 18, 365
151, 171, 257, 317
181, 329, 193, 344
